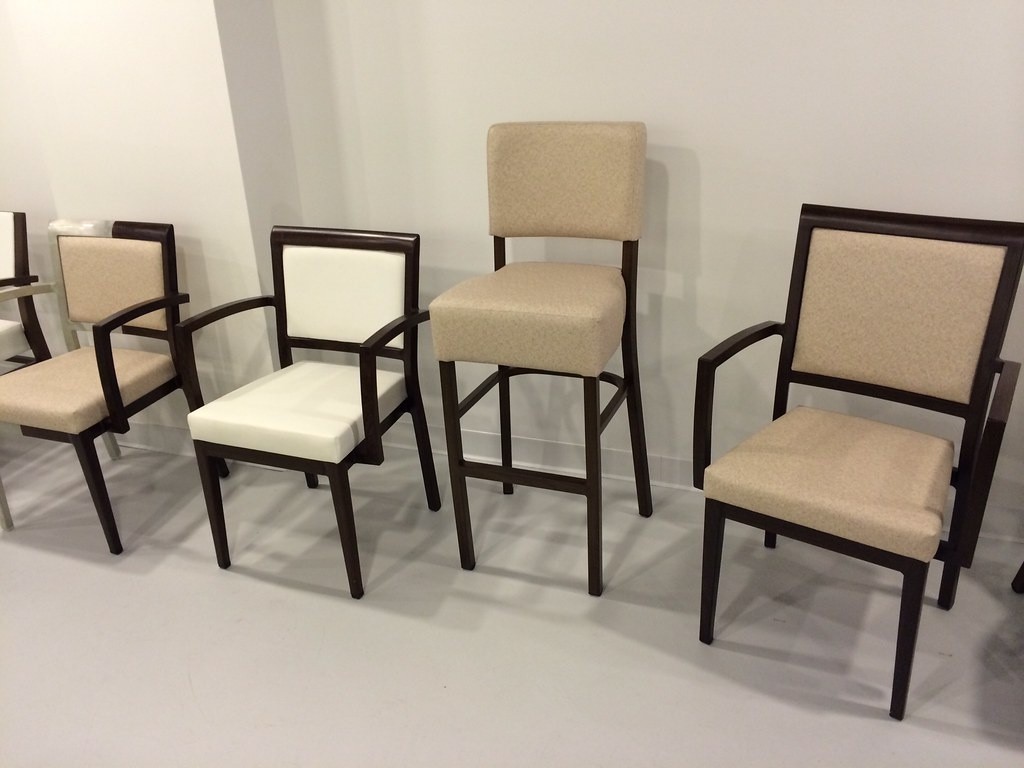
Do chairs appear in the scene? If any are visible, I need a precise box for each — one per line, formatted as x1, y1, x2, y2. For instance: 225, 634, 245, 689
174, 226, 443, 600
0, 211, 230, 554
693, 203, 1024, 720
428, 121, 654, 599
